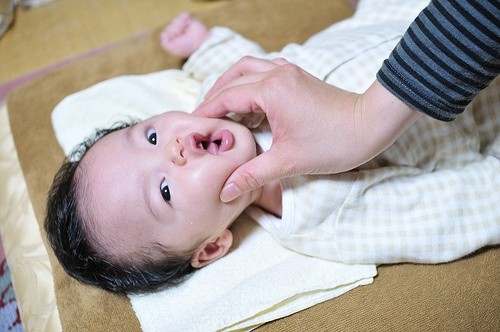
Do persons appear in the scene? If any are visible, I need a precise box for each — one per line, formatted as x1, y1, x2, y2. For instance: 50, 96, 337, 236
188, 0, 500, 203
43, 0, 500, 294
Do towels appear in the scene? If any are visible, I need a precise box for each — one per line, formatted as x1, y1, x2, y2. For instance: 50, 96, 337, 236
50, 68, 378, 332
